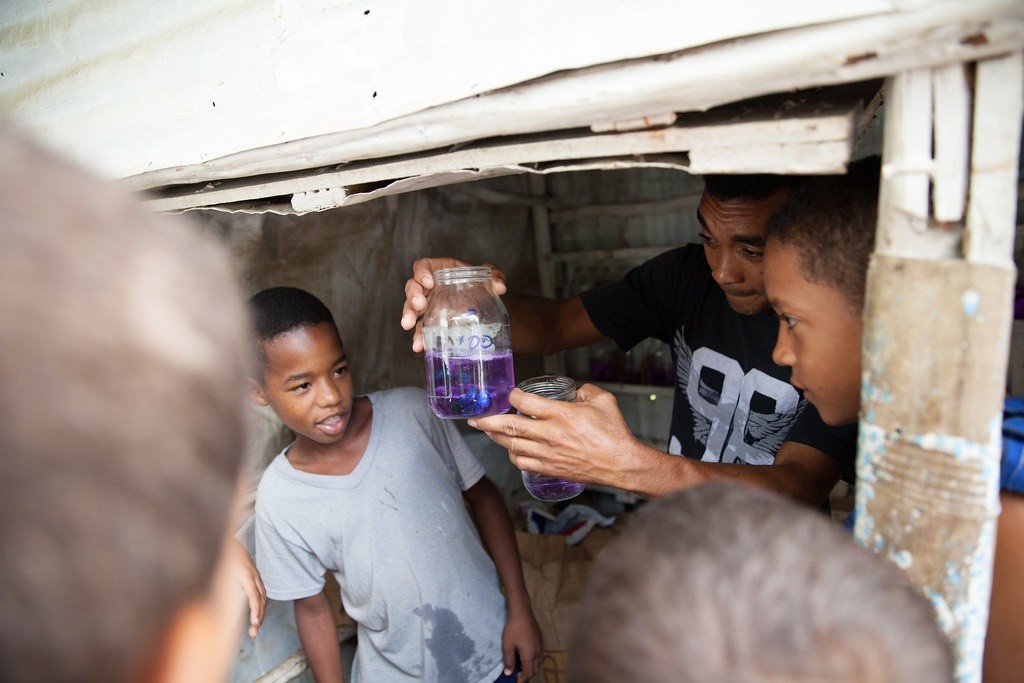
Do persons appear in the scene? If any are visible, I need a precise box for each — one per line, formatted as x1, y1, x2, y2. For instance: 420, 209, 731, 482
764, 156, 1024, 683
401, 174, 856, 519
566, 481, 954, 683
246, 286, 543, 683
0, 118, 259, 683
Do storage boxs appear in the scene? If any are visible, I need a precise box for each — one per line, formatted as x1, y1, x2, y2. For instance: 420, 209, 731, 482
574, 370, 675, 443
510, 510, 636, 683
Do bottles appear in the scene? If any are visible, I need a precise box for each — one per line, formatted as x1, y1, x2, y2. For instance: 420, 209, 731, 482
424, 266, 515, 419
518, 376, 587, 502
